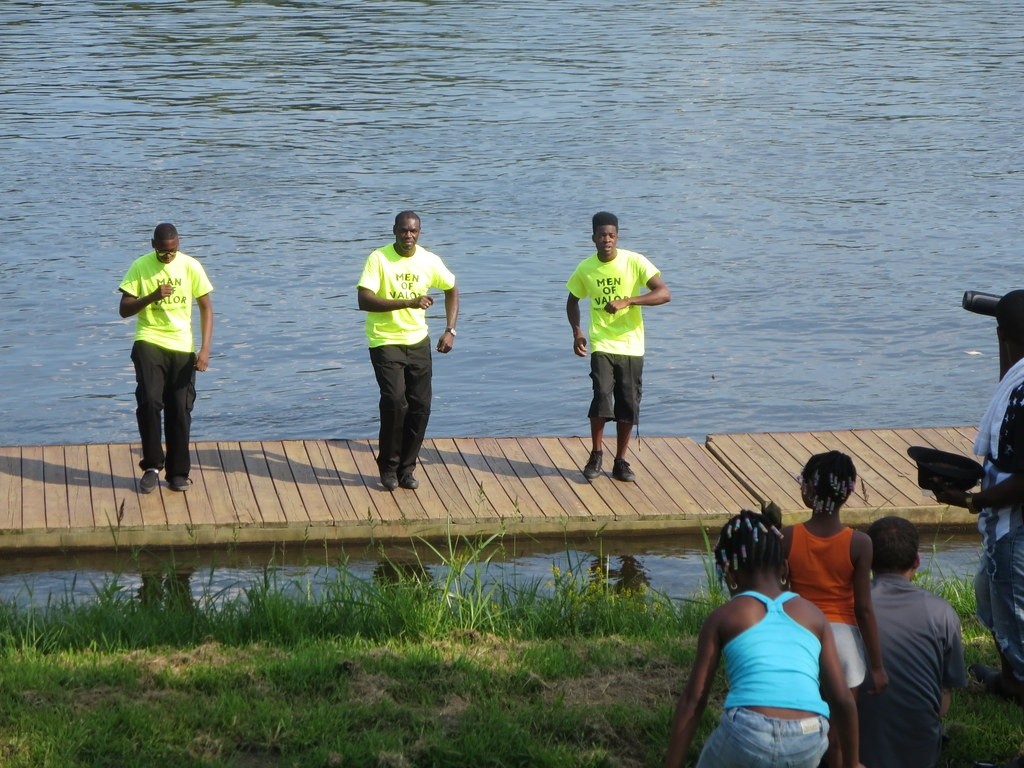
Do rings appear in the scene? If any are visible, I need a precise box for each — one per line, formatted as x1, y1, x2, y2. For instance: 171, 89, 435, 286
426, 302, 429, 306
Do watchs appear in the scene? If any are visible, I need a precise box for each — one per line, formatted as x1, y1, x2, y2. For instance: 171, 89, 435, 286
445, 327, 457, 336
966, 493, 981, 514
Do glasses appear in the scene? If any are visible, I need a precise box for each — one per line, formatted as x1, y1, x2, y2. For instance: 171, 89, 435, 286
157, 246, 177, 256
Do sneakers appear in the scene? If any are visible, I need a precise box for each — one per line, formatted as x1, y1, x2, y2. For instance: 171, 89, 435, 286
583, 451, 603, 479
165, 476, 193, 491
139, 470, 159, 493
613, 459, 635, 481
969, 664, 1024, 700
380, 471, 419, 489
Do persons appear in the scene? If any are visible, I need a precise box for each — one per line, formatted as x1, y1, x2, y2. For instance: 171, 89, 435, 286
357, 209, 459, 490
781, 450, 888, 768
565, 212, 671, 482
664, 509, 867, 768
858, 516, 967, 768
118, 222, 214, 494
906, 288, 1024, 705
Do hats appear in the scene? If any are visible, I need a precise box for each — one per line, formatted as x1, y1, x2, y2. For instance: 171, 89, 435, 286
906, 446, 986, 492
